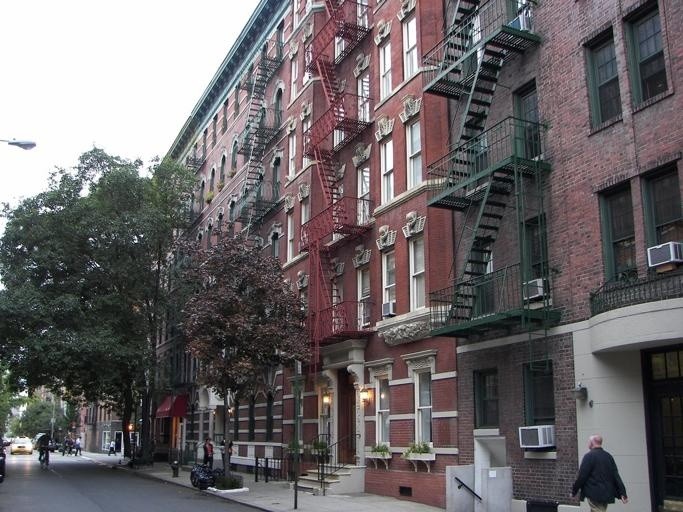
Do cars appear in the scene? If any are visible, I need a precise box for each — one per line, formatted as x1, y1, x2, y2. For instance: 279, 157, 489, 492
1, 436, 12, 483
10, 436, 33, 454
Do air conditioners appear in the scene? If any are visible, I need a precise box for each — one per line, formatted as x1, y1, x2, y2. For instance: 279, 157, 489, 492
519, 425, 555, 448
508, 16, 530, 32
522, 279, 550, 301
647, 242, 683, 267
382, 302, 396, 317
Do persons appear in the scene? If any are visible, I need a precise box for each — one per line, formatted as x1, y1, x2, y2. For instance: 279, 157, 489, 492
219, 435, 233, 470
35, 432, 53, 466
108, 439, 117, 456
49, 431, 83, 457
202, 437, 215, 469
566, 434, 629, 512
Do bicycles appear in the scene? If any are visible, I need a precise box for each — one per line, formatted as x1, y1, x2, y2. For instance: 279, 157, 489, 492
37, 445, 54, 471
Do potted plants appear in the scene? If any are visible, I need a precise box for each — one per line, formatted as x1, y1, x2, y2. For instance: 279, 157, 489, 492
401, 443, 436, 461
365, 445, 392, 459
283, 441, 331, 456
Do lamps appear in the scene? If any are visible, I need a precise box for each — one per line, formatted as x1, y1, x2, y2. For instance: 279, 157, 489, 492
322, 392, 330, 403
360, 389, 370, 406
571, 385, 588, 401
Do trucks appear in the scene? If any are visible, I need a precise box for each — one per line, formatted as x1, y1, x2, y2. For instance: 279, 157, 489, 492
34, 433, 56, 452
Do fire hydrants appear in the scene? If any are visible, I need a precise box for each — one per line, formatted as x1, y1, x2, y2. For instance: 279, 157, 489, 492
171, 458, 178, 477
